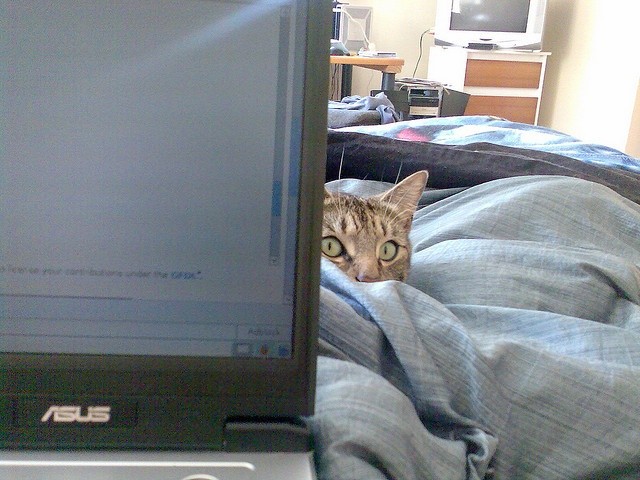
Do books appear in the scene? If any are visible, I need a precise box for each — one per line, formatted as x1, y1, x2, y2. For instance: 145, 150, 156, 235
358, 52, 397, 58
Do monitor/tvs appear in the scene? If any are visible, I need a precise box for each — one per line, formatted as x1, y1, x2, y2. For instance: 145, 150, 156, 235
434, 0, 547, 50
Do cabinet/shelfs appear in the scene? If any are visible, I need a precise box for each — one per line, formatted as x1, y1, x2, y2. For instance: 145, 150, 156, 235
426, 46, 552, 128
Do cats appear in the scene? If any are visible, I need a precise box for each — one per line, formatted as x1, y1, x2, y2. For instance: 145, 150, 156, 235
321, 147, 430, 286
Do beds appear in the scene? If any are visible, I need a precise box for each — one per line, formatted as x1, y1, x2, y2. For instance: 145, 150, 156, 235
301, 116, 639, 480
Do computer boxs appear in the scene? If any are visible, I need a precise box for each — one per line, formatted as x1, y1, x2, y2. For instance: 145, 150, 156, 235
395, 78, 443, 121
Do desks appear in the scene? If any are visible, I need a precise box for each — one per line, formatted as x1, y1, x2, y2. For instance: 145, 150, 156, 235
329, 52, 405, 100
